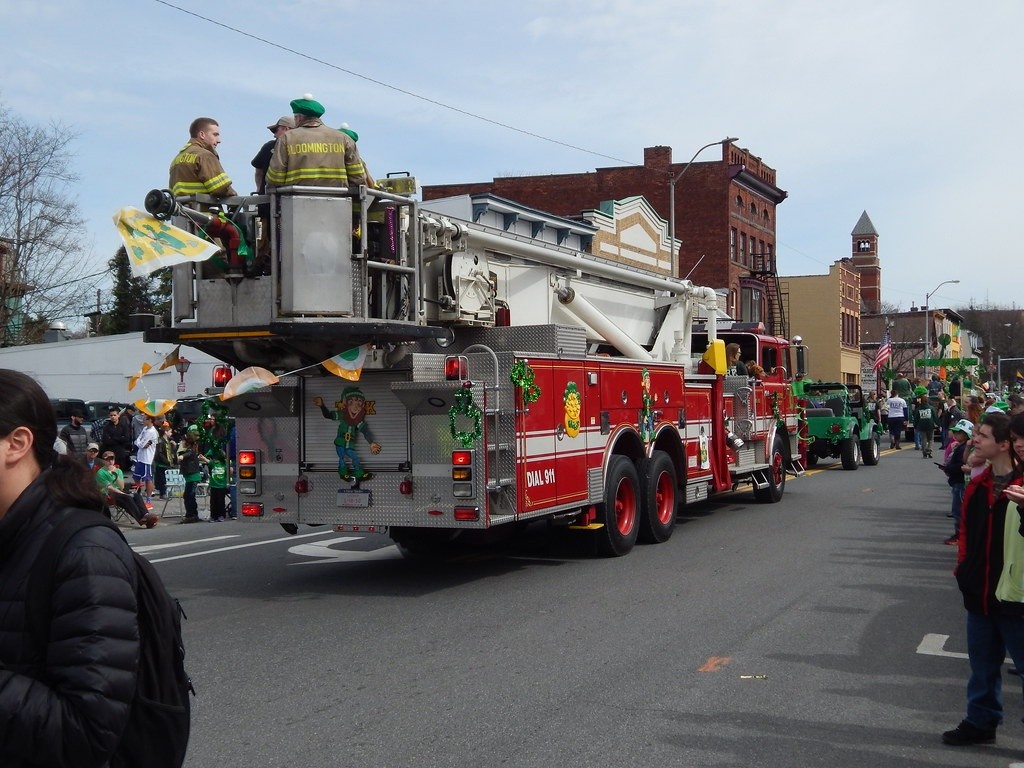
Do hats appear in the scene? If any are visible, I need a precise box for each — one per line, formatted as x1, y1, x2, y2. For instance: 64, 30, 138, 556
267, 116, 295, 128
985, 401, 1010, 414
102, 451, 115, 459
337, 123, 358, 142
949, 420, 975, 438
290, 93, 325, 118
126, 405, 136, 411
87, 443, 99, 450
71, 409, 84, 416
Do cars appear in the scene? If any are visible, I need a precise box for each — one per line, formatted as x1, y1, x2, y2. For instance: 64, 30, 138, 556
802, 382, 880, 471
984, 391, 1007, 405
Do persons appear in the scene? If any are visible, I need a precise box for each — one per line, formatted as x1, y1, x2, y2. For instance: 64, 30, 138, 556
867, 391, 880, 424
81, 443, 102, 471
854, 392, 869, 424
265, 94, 373, 234
879, 396, 889, 433
101, 408, 132, 470
928, 374, 1024, 745
884, 389, 908, 450
892, 373, 911, 422
0, 368, 148, 768
95, 451, 159, 528
251, 116, 295, 219
912, 398, 922, 450
170, 118, 238, 212
913, 396, 940, 458
726, 343, 748, 375
126, 405, 233, 523
59, 410, 89, 456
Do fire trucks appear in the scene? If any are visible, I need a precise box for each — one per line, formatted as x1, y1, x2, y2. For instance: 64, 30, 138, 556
128, 170, 810, 575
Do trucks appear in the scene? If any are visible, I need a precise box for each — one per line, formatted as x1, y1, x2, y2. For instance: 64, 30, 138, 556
904, 395, 961, 442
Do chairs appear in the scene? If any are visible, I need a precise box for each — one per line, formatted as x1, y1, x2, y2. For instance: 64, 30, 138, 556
807, 398, 816, 409
825, 397, 844, 417
161, 469, 209, 518
225, 492, 232, 518
92, 464, 144, 524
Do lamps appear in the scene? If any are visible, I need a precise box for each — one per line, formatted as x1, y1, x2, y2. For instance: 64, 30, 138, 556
175, 356, 191, 383
48, 320, 66, 331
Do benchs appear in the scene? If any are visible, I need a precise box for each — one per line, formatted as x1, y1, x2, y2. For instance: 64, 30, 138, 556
806, 408, 835, 417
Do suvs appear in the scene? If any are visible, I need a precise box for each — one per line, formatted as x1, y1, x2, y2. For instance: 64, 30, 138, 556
170, 394, 214, 441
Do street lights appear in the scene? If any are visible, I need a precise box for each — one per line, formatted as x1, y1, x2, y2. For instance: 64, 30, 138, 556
989, 323, 1012, 393
670, 136, 740, 277
924, 279, 961, 380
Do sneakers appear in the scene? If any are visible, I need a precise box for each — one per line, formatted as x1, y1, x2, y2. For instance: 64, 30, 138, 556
146, 513, 160, 528
941, 719, 996, 744
139, 518, 157, 527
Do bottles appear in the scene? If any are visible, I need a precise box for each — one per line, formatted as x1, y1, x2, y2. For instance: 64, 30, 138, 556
147, 496, 151, 503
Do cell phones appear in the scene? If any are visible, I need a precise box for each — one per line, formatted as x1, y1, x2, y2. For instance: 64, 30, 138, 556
147, 440, 152, 445
934, 462, 944, 467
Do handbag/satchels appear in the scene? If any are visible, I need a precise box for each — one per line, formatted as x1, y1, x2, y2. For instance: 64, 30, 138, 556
876, 422, 883, 435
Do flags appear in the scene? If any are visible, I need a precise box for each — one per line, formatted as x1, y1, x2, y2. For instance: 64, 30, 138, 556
872, 324, 891, 373
1017, 371, 1024, 383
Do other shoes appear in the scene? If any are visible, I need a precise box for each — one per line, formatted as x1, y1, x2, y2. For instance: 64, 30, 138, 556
946, 512, 954, 519
890, 442, 946, 458
210, 518, 214, 522
944, 534, 958, 545
1008, 667, 1018, 674
216, 516, 224, 521
180, 516, 199, 524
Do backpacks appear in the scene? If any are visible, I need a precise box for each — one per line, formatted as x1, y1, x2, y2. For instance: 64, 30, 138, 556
28, 512, 196, 768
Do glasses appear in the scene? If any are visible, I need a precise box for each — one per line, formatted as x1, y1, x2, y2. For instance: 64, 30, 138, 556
90, 449, 98, 453
738, 352, 741, 355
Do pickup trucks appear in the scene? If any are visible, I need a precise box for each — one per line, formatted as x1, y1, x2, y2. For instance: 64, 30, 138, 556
48, 398, 130, 446
